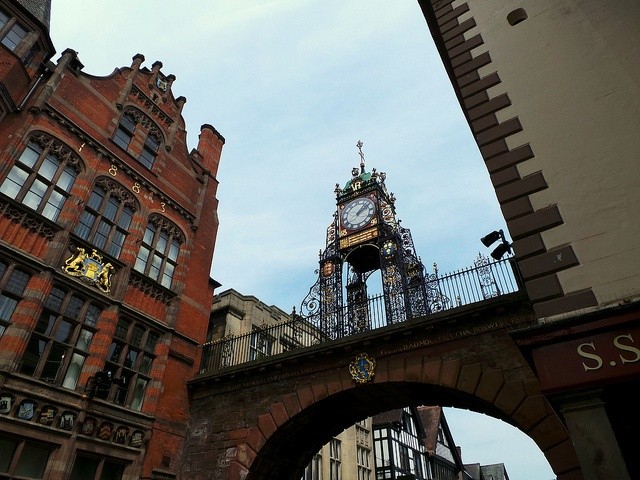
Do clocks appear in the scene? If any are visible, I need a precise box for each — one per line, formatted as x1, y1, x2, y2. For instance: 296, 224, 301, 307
341, 197, 376, 230
